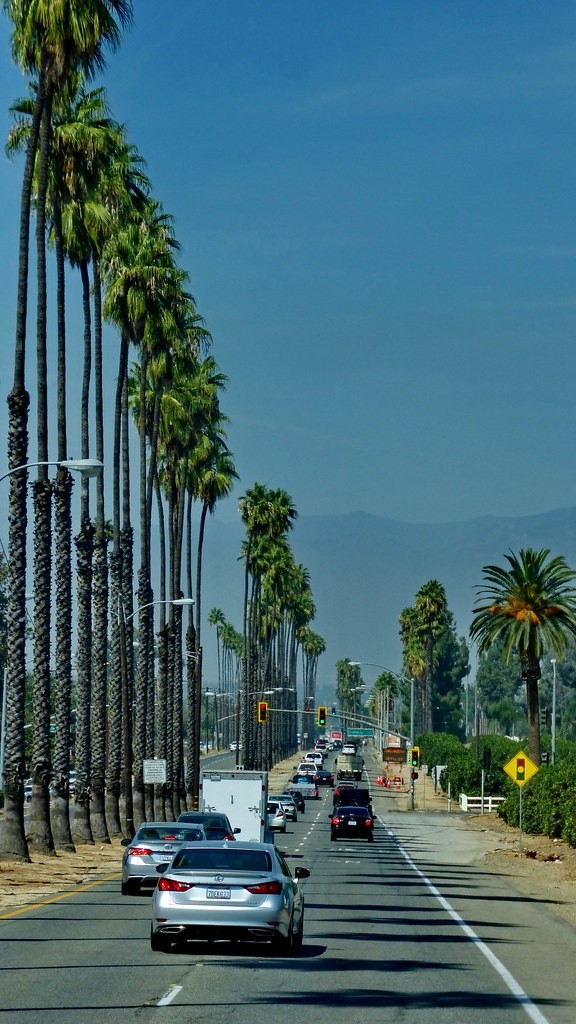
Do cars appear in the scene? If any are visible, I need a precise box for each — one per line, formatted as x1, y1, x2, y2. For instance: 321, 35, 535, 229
327, 805, 378, 842
148, 840, 311, 957
24, 770, 78, 802
174, 809, 242, 841
267, 800, 289, 832
268, 728, 374, 822
119, 820, 210, 896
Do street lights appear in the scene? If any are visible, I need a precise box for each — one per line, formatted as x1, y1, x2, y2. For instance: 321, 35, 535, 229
347, 661, 416, 811
349, 684, 390, 762
205, 687, 296, 768
117, 591, 195, 840
550, 658, 556, 766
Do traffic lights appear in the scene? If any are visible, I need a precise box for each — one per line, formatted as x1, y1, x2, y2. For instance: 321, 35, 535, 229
410, 748, 419, 769
411, 772, 418, 780
317, 705, 327, 727
259, 702, 268, 723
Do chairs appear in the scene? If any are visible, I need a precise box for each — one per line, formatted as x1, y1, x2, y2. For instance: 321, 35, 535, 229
189, 851, 213, 868
146, 829, 160, 839
210, 821, 221, 827
184, 833, 199, 841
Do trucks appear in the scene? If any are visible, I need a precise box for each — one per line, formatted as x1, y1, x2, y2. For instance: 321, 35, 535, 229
198, 768, 270, 844
